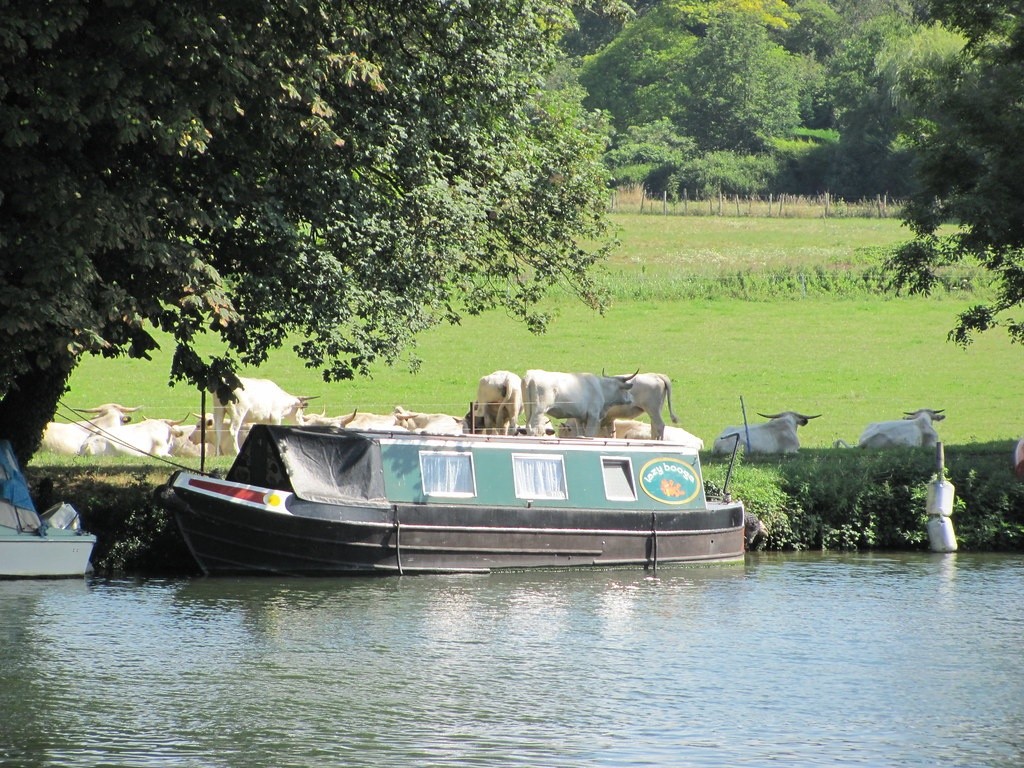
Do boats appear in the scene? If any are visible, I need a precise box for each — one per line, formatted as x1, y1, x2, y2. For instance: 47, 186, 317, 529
152, 420, 749, 578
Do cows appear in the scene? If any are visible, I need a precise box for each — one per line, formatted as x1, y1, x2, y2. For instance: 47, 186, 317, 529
33, 367, 705, 460
834, 408, 946, 452
712, 412, 823, 465
